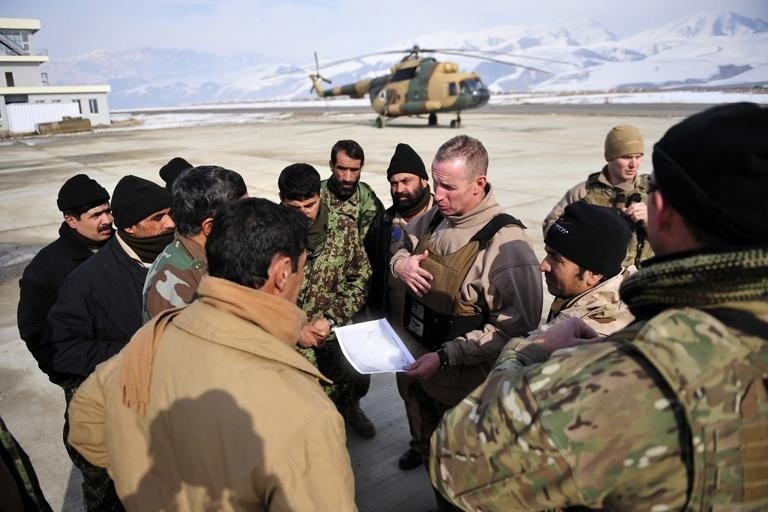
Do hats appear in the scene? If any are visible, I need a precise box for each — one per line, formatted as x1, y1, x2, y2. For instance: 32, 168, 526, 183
159, 157, 192, 185
57, 174, 110, 212
111, 175, 172, 229
386, 143, 429, 181
604, 124, 644, 161
544, 200, 632, 279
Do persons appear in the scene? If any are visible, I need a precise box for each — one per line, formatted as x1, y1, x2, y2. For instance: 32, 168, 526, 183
67, 198, 359, 512
1, 419, 53, 512
426, 99, 763, 512
17, 156, 247, 512
277, 135, 544, 512
539, 125, 658, 339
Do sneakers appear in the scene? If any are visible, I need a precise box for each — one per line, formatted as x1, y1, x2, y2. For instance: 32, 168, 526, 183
342, 400, 375, 438
398, 448, 422, 470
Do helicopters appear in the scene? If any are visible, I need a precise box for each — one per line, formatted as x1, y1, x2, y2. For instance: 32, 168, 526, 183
261, 41, 574, 128
